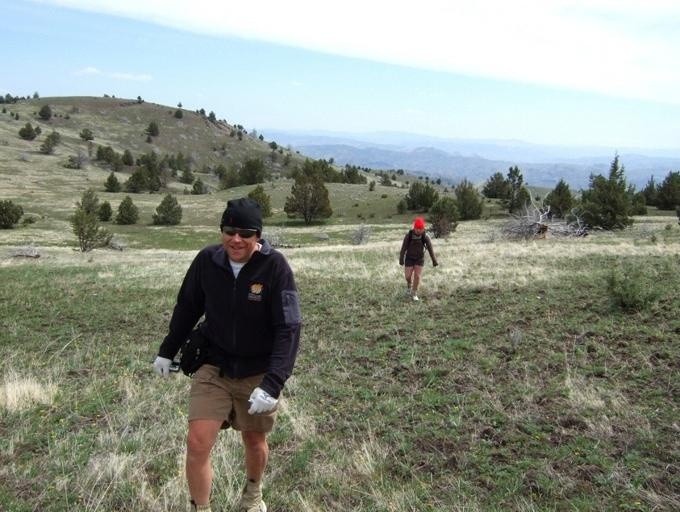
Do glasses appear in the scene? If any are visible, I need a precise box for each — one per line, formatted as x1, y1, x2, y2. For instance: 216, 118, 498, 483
221, 226, 258, 238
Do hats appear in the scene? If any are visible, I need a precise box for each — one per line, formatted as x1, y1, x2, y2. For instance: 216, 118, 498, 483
220, 197, 262, 237
414, 218, 425, 229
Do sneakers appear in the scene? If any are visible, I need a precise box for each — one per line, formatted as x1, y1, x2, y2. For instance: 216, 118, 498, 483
412, 296, 419, 300
406, 288, 411, 295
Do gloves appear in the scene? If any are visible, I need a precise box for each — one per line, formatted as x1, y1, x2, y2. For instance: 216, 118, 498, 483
153, 356, 172, 377
247, 386, 278, 416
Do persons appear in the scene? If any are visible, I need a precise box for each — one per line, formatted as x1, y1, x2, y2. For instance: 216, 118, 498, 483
399, 217, 439, 300
153, 196, 302, 512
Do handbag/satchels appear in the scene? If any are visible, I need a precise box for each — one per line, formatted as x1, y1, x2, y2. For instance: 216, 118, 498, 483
180, 322, 217, 376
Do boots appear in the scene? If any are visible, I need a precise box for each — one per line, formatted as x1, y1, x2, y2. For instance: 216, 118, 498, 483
243, 479, 267, 512
